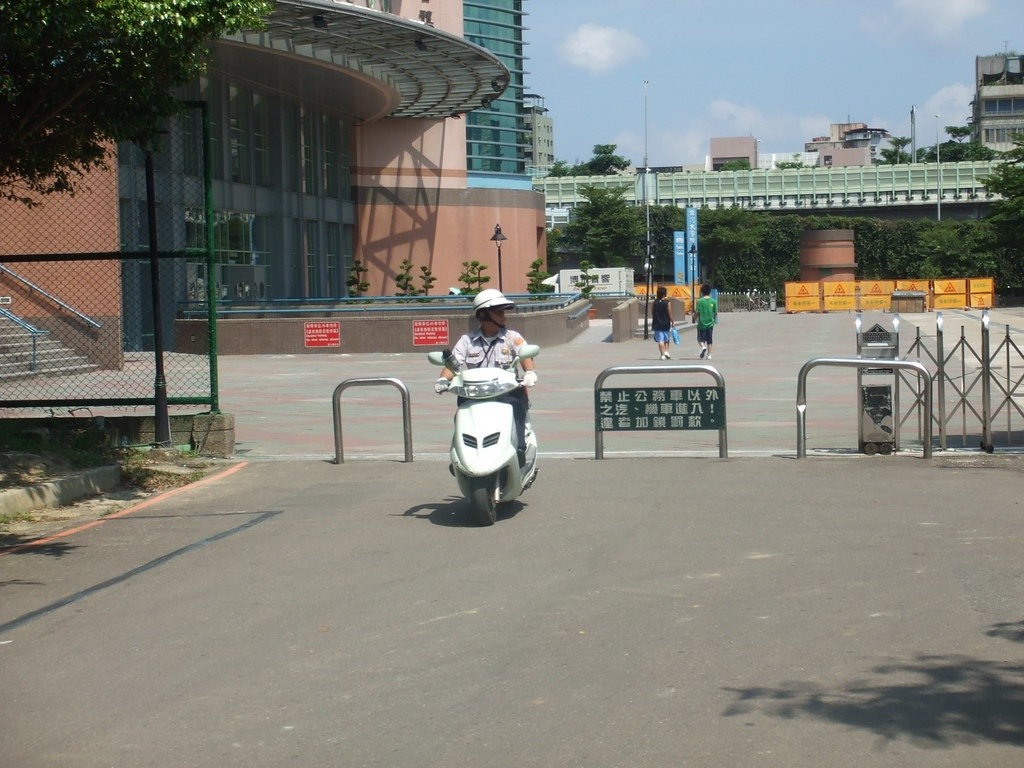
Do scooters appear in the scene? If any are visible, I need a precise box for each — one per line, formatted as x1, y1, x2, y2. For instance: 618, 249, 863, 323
427, 345, 540, 525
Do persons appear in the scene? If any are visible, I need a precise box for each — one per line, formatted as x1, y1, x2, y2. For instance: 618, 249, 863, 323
652, 287, 675, 359
435, 290, 537, 477
692, 285, 718, 360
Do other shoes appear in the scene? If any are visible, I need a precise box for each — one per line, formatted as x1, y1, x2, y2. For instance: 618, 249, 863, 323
700, 347, 706, 358
660, 352, 671, 360
707, 354, 712, 359
449, 464, 455, 476
517, 448, 526, 468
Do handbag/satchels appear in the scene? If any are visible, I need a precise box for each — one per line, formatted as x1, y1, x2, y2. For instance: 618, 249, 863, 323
672, 327, 680, 345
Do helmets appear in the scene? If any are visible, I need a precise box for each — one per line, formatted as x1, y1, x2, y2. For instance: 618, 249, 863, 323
753, 289, 757, 291
473, 288, 515, 313
747, 289, 750, 291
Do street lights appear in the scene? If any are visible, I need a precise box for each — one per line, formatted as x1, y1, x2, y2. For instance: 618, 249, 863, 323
642, 80, 651, 285
935, 114, 940, 220
641, 233, 657, 340
688, 242, 698, 324
490, 223, 509, 294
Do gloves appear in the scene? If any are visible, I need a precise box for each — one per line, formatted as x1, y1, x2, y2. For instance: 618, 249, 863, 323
435, 377, 449, 393
524, 371, 538, 387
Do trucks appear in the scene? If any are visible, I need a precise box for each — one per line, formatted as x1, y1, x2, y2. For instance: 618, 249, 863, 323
542, 268, 634, 297
